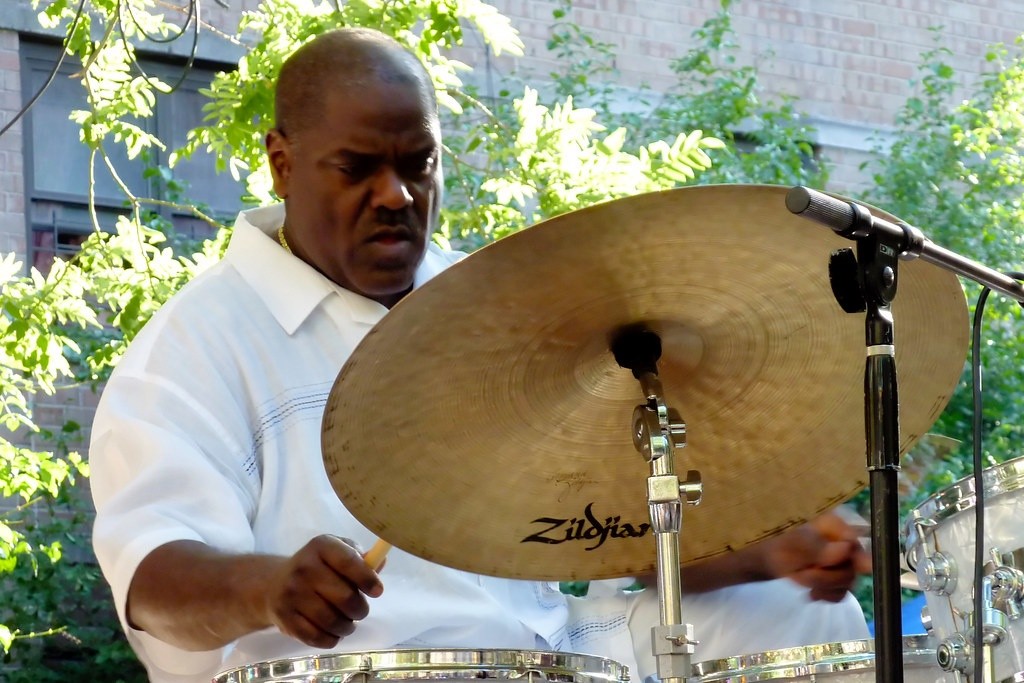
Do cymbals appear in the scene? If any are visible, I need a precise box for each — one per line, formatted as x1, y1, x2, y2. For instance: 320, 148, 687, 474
317, 181, 973, 585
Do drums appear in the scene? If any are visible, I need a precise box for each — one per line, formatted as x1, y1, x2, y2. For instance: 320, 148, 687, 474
216, 648, 629, 683
695, 635, 977, 683
904, 456, 1024, 683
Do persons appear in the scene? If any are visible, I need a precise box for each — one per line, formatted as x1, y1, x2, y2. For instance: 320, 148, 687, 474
90, 26, 872, 683
